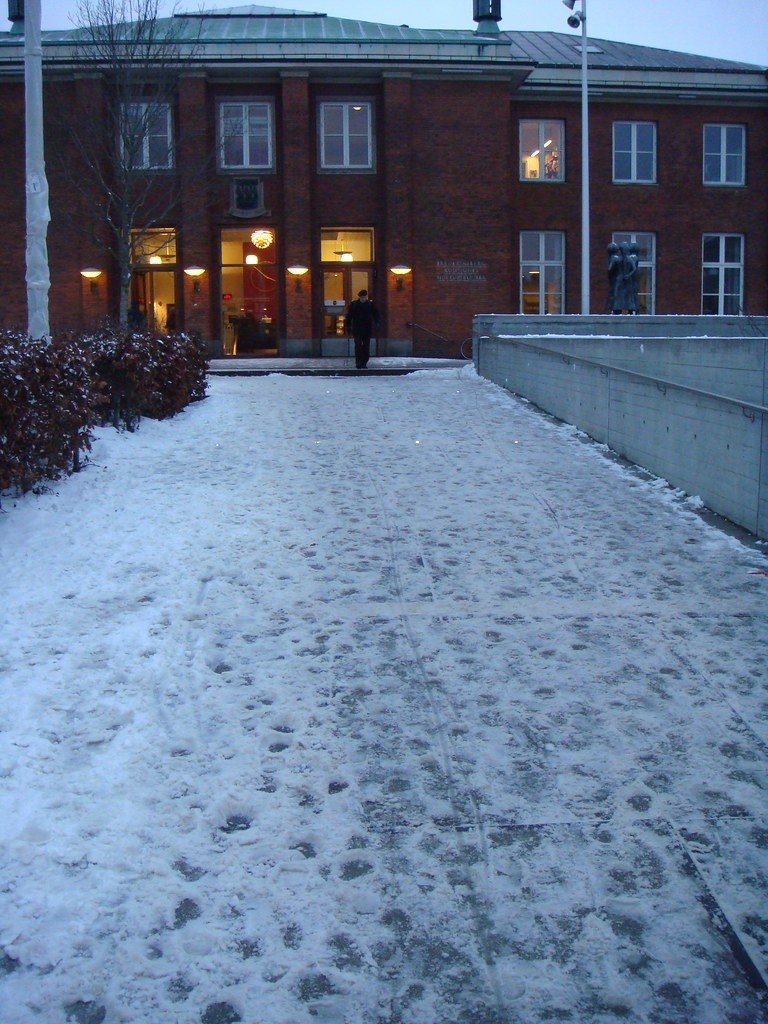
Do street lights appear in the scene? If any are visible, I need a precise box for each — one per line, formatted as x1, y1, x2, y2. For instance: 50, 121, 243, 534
568, 11, 589, 315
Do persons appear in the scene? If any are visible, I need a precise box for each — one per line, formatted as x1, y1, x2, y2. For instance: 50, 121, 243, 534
607, 240, 640, 314
343, 290, 382, 370
163, 307, 175, 337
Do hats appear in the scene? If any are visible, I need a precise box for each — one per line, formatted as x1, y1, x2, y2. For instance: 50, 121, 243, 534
358, 290, 367, 296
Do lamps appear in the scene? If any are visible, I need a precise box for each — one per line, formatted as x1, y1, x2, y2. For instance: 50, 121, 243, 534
390, 265, 412, 291
183, 264, 206, 294
287, 264, 308, 293
78, 265, 101, 292
250, 230, 273, 249
